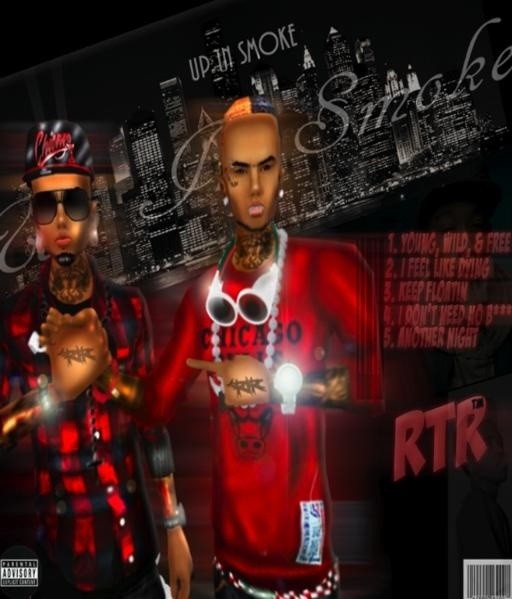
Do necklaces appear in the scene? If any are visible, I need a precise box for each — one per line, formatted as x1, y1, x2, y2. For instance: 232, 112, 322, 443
211, 226, 289, 411
39, 279, 112, 470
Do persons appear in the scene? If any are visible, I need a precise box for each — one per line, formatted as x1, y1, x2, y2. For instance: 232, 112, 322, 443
1, 120, 200, 599
38, 110, 388, 599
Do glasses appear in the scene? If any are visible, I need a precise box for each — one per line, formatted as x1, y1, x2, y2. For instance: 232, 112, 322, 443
32, 190, 91, 225
207, 266, 280, 327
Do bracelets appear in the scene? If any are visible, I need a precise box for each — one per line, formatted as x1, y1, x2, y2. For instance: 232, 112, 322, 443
160, 500, 188, 531
270, 364, 302, 416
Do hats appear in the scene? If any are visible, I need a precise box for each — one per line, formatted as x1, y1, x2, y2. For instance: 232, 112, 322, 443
25, 119, 95, 180
223, 95, 277, 119
